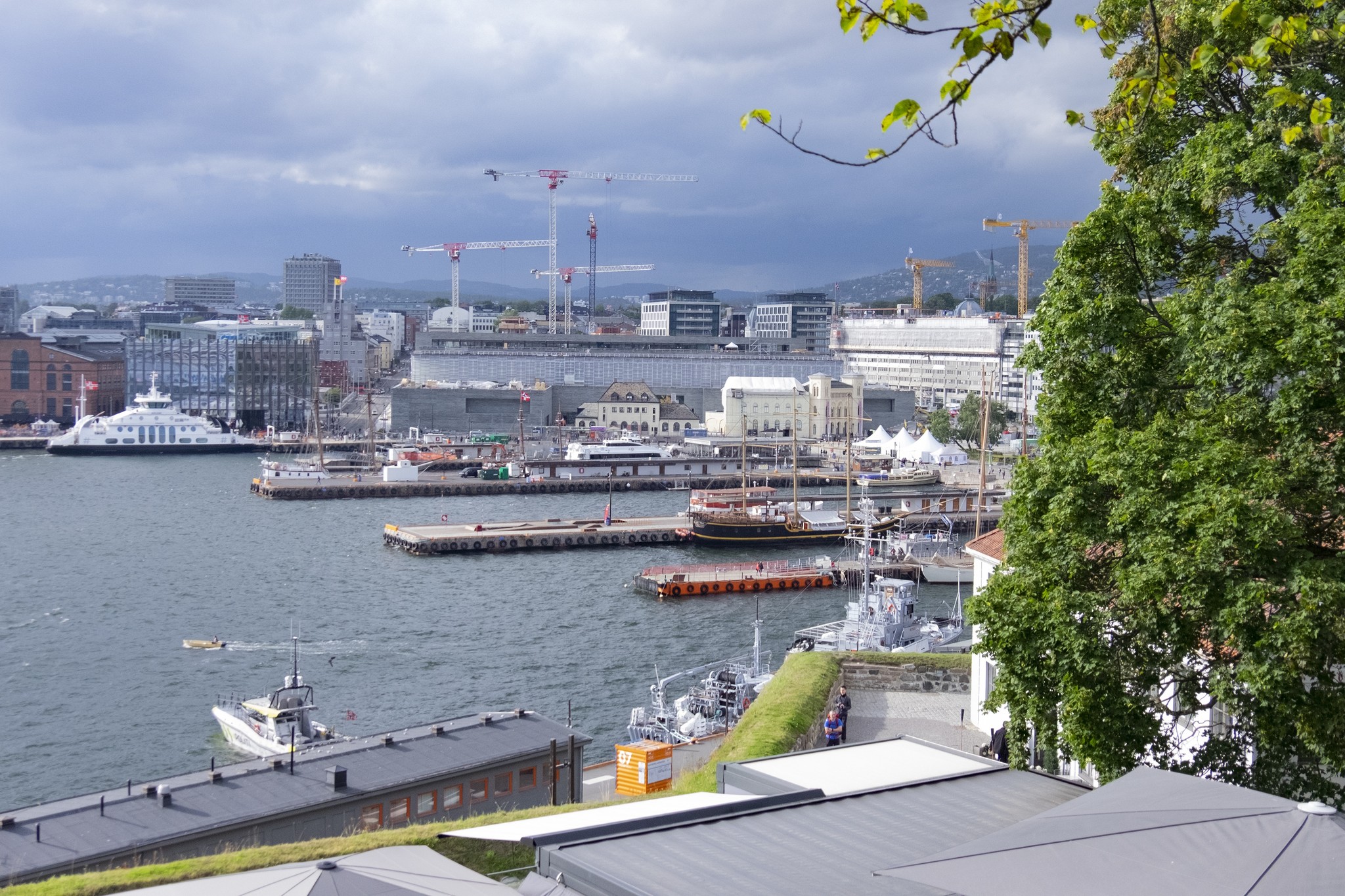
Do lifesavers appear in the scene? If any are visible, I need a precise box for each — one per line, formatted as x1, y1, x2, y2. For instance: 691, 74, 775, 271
743, 697, 750, 709
888, 604, 896, 613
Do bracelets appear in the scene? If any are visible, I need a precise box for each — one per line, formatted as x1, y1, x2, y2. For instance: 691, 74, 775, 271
831, 728, 833, 732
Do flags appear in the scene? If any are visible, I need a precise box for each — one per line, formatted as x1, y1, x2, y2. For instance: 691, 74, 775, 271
238, 314, 250, 325
335, 278, 341, 285
520, 390, 531, 401
85, 380, 99, 392
340, 276, 348, 282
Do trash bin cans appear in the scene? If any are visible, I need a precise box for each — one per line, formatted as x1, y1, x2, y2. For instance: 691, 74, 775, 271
498, 467, 509, 479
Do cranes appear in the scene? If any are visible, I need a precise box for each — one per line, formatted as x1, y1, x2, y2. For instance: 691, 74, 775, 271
905, 246, 956, 309
402, 168, 699, 334
982, 211, 1082, 319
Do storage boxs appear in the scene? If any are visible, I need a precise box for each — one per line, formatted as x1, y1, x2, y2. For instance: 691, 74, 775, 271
615, 738, 673, 797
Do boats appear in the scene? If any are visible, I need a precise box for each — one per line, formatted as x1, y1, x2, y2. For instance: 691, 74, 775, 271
565, 440, 672, 460
183, 639, 228, 649
627, 500, 964, 745
212, 619, 348, 759
921, 564, 974, 583
45, 371, 270, 454
257, 456, 331, 479
857, 467, 939, 486
687, 386, 960, 546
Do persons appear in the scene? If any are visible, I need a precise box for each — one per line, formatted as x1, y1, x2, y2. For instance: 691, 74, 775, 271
824, 710, 843, 749
750, 545, 908, 577
989, 721, 1011, 763
834, 685, 852, 743
0, 415, 1031, 484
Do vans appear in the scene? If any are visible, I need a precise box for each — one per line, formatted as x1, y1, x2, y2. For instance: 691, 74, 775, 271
459, 467, 482, 478
